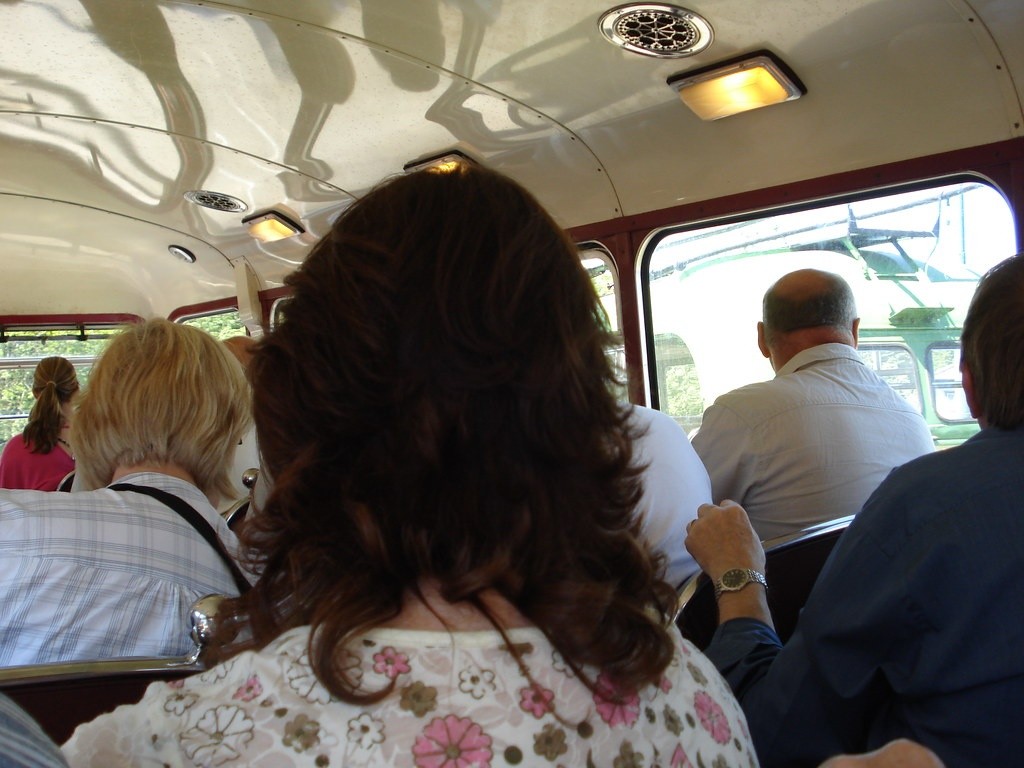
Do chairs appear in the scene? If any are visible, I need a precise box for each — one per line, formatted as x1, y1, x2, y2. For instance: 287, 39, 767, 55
221, 468, 260, 525
56, 452, 75, 493
0, 591, 242, 725
663, 512, 857, 650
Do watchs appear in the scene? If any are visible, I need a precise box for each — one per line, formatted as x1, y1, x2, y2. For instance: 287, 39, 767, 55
715, 568, 768, 601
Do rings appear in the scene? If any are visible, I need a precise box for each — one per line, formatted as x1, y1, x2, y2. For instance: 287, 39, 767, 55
691, 519, 696, 527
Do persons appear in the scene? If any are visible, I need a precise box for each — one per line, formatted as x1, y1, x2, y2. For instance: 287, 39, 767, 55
684, 255, 1024, 768
621, 403, 710, 594
691, 269, 937, 540
63, 165, 758, 768
0, 357, 80, 491
0, 322, 259, 664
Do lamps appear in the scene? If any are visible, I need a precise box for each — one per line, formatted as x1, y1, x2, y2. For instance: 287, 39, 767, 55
666, 49, 808, 123
241, 210, 306, 242
403, 150, 479, 174
